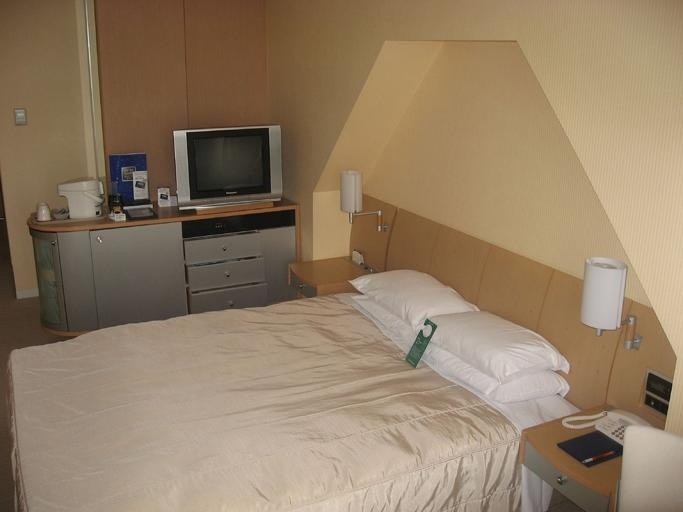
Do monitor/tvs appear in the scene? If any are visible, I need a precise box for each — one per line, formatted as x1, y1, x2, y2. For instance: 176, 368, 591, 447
173, 124, 283, 215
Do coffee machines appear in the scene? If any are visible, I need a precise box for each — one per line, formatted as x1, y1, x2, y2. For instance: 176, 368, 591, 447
56, 178, 104, 218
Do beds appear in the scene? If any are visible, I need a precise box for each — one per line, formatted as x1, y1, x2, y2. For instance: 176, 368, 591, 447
7, 209, 633, 512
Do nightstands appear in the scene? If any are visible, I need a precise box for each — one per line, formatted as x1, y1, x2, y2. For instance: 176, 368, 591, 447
520, 403, 621, 512
288, 255, 370, 298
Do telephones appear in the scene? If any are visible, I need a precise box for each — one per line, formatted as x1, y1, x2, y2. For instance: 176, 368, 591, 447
594, 409, 654, 447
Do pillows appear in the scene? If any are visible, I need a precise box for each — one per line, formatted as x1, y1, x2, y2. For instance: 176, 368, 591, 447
348, 269, 570, 405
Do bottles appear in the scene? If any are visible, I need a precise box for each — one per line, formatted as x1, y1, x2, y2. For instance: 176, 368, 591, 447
107, 181, 122, 214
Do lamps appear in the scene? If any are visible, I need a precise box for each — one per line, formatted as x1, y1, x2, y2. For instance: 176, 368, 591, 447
338, 168, 389, 234
578, 256, 643, 351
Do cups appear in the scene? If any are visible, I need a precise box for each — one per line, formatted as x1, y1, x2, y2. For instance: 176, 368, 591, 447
35, 201, 50, 222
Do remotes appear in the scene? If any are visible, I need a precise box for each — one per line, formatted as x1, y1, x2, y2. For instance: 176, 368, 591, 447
406, 319, 437, 368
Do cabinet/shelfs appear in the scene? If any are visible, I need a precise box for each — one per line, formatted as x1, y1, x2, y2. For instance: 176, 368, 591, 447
29, 209, 298, 337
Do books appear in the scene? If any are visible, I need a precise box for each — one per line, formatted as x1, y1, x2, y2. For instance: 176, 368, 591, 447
108, 152, 149, 202
107, 199, 153, 212
